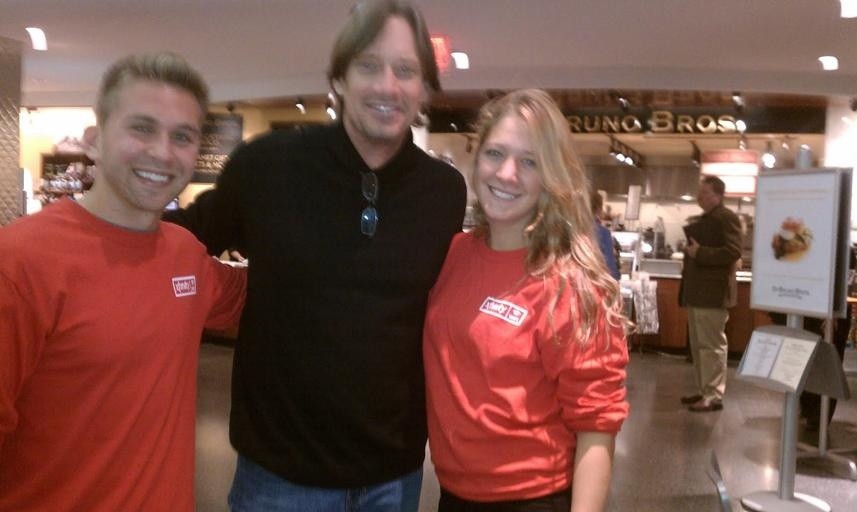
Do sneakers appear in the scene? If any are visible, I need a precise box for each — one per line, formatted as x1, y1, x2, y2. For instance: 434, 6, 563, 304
680, 393, 723, 411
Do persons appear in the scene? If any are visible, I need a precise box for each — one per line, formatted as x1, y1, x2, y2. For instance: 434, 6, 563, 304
589, 190, 616, 277
678, 175, 745, 411
2, 53, 249, 511
797, 302, 852, 432
418, 88, 639, 512
154, 0, 461, 510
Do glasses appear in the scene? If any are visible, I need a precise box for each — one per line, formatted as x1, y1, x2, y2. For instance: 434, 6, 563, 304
360, 170, 379, 236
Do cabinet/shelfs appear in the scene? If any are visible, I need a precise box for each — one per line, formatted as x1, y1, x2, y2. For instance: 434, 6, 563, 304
41, 153, 95, 209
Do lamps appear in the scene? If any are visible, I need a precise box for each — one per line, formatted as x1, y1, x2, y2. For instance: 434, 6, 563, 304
607, 135, 646, 170
689, 140, 702, 168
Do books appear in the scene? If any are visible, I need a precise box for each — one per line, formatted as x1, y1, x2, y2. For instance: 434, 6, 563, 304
680, 218, 725, 248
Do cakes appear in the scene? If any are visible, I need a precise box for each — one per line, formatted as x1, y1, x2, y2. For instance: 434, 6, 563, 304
772, 215, 815, 262
